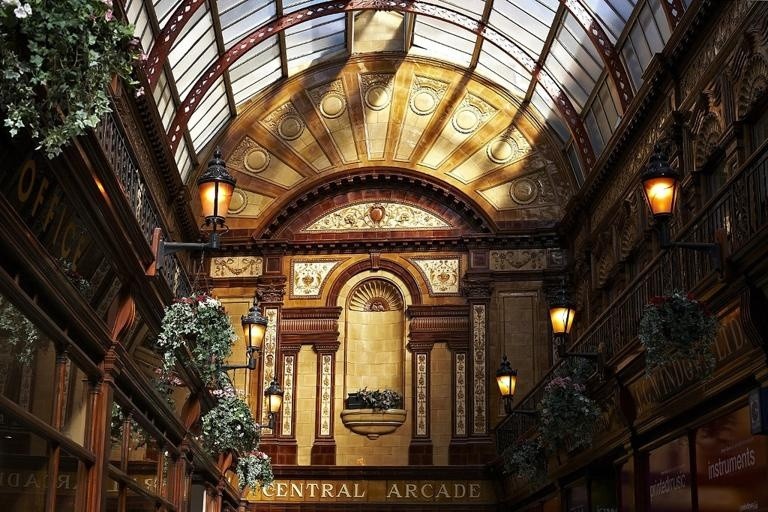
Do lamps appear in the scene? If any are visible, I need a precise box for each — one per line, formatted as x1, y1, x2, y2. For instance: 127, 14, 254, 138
497, 355, 537, 415
547, 289, 600, 359
223, 293, 268, 370
258, 376, 285, 429
161, 146, 238, 249
639, 150, 718, 252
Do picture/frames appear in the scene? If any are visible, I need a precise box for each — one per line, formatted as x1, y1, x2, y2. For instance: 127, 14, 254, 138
748, 387, 765, 436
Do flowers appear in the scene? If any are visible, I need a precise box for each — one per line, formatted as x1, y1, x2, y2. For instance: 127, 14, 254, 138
110, 402, 145, 449
504, 435, 548, 483
534, 377, 604, 450
154, 359, 180, 408
637, 291, 716, 373
2, 0, 149, 162
1, 299, 39, 368
196, 380, 262, 454
159, 290, 239, 386
236, 448, 274, 495
358, 384, 403, 412
57, 257, 89, 296
